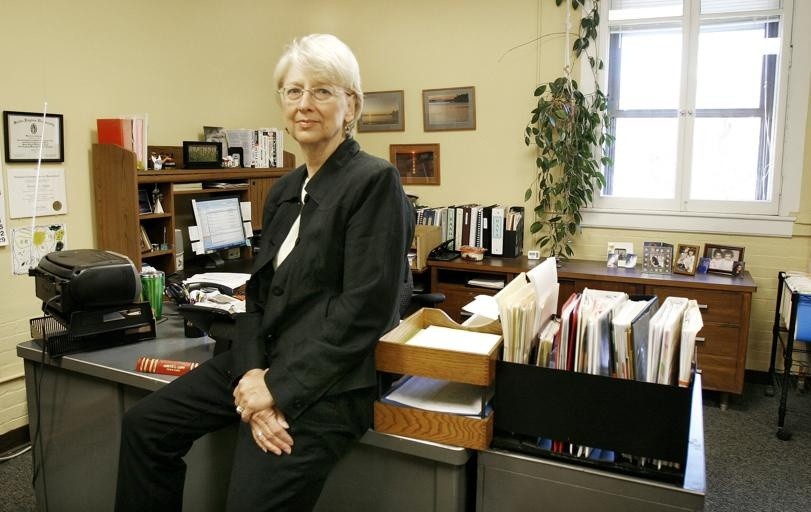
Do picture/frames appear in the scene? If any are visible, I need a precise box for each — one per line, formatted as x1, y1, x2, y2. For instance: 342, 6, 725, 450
181, 140, 221, 169
388, 143, 441, 187
672, 243, 701, 276
2, 107, 68, 167
420, 83, 477, 134
606, 241, 634, 268
641, 245, 672, 276
702, 242, 746, 278
352, 88, 406, 134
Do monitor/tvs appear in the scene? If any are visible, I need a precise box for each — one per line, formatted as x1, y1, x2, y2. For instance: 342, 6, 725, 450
191, 194, 246, 255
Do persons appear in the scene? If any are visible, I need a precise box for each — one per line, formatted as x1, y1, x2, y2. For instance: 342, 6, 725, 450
677, 247, 742, 276
115, 34, 417, 511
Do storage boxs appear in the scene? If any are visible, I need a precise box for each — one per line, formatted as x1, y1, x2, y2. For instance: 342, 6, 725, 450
493, 348, 698, 491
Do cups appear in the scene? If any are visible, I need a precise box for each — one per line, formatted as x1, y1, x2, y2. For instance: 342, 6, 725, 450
142, 271, 166, 320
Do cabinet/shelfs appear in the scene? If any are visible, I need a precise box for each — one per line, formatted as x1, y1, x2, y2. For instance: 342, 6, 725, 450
643, 276, 759, 412
766, 269, 811, 445
424, 259, 510, 324
89, 137, 297, 284
471, 365, 710, 511
510, 265, 643, 317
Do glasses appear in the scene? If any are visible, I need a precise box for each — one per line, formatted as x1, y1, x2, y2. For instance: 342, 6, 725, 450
277, 85, 352, 100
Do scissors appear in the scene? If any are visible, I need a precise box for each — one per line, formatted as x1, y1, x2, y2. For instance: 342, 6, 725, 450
164, 282, 188, 304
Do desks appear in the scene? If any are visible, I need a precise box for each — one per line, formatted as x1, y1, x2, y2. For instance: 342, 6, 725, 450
12, 335, 473, 512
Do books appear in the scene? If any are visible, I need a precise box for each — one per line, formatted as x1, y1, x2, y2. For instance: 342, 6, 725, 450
226, 126, 283, 168
415, 205, 522, 255
97, 114, 148, 171
495, 256, 703, 472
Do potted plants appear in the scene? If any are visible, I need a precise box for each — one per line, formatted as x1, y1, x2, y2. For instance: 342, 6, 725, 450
520, 0, 617, 267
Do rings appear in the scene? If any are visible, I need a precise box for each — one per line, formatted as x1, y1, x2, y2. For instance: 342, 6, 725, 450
257, 432, 262, 437
236, 406, 244, 415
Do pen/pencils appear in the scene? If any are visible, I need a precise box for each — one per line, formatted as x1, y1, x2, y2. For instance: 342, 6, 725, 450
181, 283, 207, 304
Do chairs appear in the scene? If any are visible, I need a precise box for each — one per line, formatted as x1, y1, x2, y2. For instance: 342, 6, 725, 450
396, 255, 445, 319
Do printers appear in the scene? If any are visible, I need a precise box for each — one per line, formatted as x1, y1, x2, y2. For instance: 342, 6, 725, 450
30, 249, 141, 310
